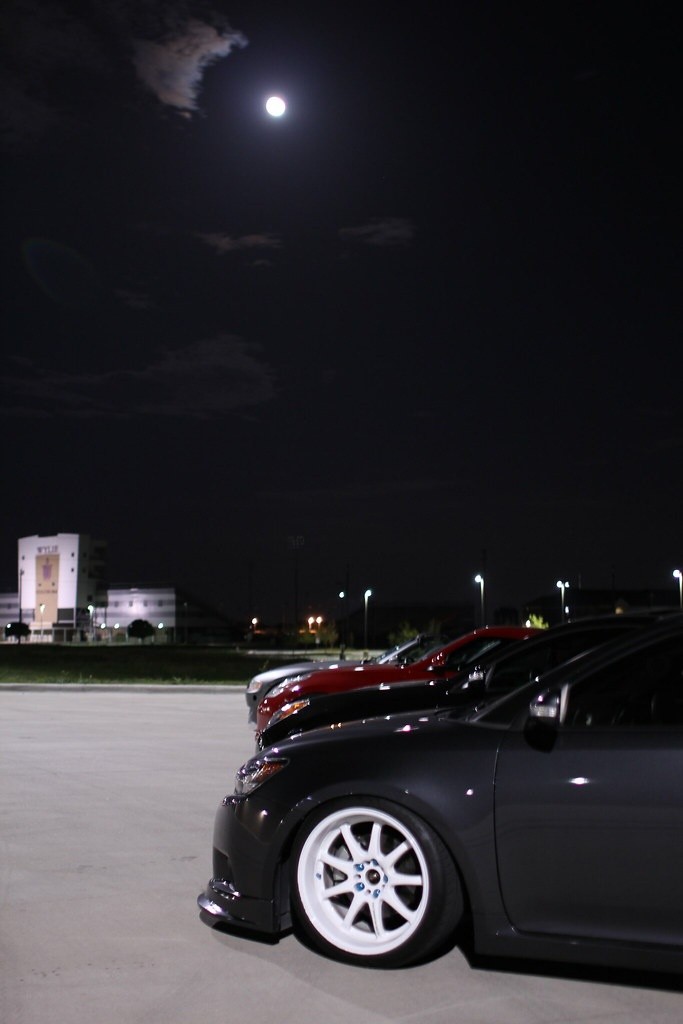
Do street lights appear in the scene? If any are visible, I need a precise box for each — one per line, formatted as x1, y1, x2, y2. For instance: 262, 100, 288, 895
556, 580, 566, 613
474, 574, 485, 609
671, 569, 683, 611
363, 588, 374, 640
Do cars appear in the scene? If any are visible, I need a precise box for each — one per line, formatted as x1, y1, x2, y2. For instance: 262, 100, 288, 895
256, 624, 546, 745
197, 612, 681, 980
246, 629, 459, 725
252, 609, 681, 754
